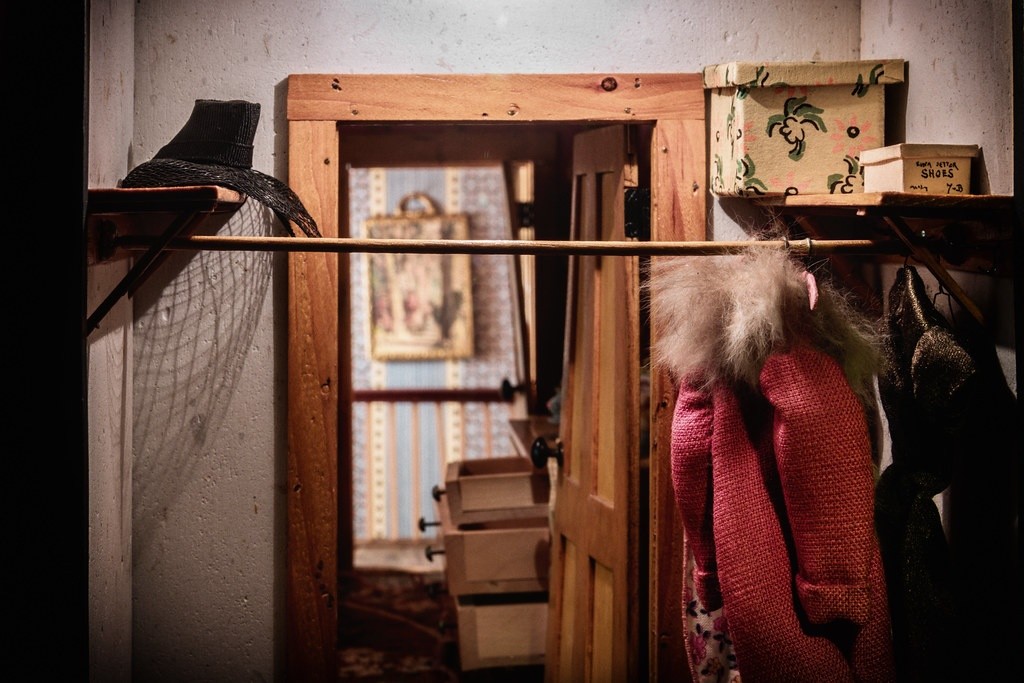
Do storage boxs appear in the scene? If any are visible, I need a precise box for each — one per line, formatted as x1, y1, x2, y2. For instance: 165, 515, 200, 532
858, 145, 979, 195
702, 60, 905, 197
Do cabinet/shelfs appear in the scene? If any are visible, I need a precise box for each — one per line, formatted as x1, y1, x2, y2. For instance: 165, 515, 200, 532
419, 416, 558, 673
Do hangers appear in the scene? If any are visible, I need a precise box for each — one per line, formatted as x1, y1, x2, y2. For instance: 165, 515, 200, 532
777, 234, 967, 372
